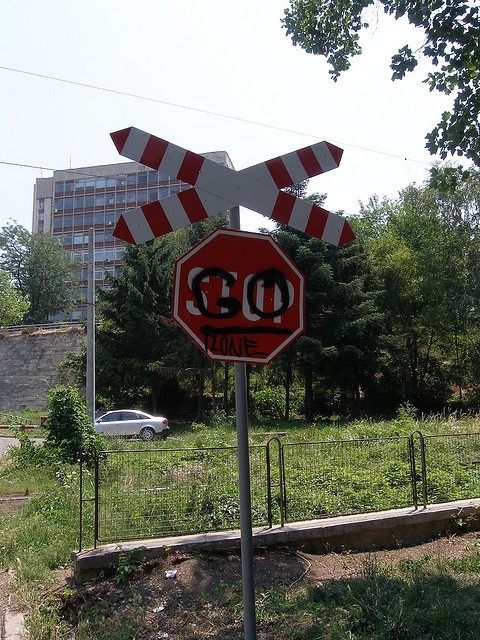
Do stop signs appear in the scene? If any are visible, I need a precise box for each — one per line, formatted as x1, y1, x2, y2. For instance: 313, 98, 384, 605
171, 227, 307, 365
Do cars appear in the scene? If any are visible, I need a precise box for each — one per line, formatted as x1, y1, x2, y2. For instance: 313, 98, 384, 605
94, 411, 170, 442
